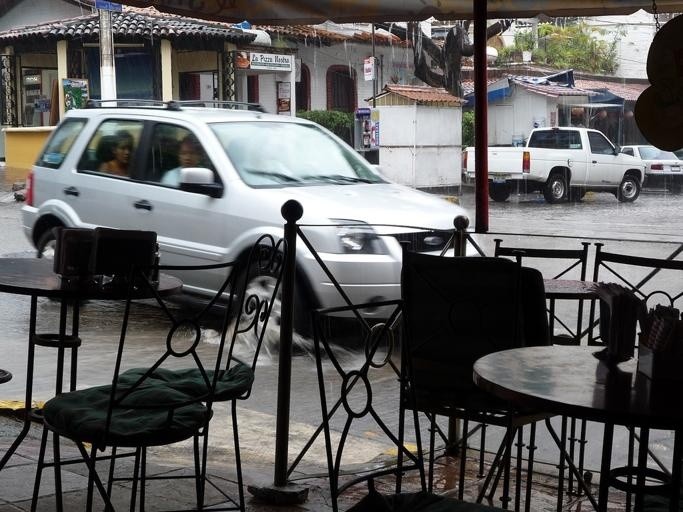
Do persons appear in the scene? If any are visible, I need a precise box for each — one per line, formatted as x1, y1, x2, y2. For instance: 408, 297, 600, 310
177, 134, 204, 167
98, 130, 134, 176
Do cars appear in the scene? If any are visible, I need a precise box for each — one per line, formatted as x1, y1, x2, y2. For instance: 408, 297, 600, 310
614, 145, 683, 194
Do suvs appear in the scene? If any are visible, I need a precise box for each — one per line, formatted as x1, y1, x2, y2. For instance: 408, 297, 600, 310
21, 97, 474, 350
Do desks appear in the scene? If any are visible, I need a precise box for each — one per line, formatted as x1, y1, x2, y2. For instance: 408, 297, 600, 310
0, 257, 183, 512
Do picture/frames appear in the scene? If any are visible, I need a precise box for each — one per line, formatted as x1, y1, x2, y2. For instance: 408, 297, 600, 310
62, 78, 90, 113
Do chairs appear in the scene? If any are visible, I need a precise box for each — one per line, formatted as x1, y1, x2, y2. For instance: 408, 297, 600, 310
395, 237, 682, 512
105, 236, 288, 509
89, 135, 115, 170
31, 261, 240, 512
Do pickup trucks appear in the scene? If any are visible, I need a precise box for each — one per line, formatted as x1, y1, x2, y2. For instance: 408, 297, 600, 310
463, 127, 646, 204
